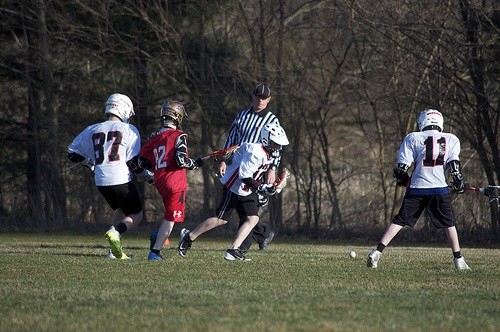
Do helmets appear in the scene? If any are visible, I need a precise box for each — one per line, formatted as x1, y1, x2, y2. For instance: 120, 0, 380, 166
104, 94, 135, 122
260, 124, 290, 157
161, 101, 188, 128
418, 109, 443, 132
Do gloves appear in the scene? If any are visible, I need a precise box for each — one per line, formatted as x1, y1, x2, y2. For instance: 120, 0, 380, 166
257, 193, 268, 207
81, 158, 95, 172
257, 184, 277, 196
186, 158, 204, 169
134, 170, 155, 185
396, 174, 410, 186
451, 182, 464, 193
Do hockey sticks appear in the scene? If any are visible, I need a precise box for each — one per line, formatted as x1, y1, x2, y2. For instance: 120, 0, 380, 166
257, 168, 288, 207
82, 157, 155, 184
194, 144, 238, 160
464, 186, 500, 201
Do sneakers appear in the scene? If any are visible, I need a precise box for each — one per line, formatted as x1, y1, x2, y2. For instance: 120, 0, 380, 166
367, 249, 381, 269
239, 247, 248, 255
104, 225, 123, 258
108, 249, 131, 259
224, 249, 252, 261
150, 229, 160, 248
259, 232, 274, 250
453, 256, 472, 271
177, 227, 194, 257
148, 253, 164, 260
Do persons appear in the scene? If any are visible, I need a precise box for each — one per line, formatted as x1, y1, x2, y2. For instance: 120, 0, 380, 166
218, 84, 280, 256
366, 109, 472, 272
179, 123, 289, 261
66, 93, 152, 260
140, 100, 205, 260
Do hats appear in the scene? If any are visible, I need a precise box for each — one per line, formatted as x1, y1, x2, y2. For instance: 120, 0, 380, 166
254, 84, 271, 97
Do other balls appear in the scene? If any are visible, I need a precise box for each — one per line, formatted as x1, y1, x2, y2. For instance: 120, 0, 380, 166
349, 251, 356, 258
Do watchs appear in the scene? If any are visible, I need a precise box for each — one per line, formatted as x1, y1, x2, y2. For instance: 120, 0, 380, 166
269, 166, 276, 170
